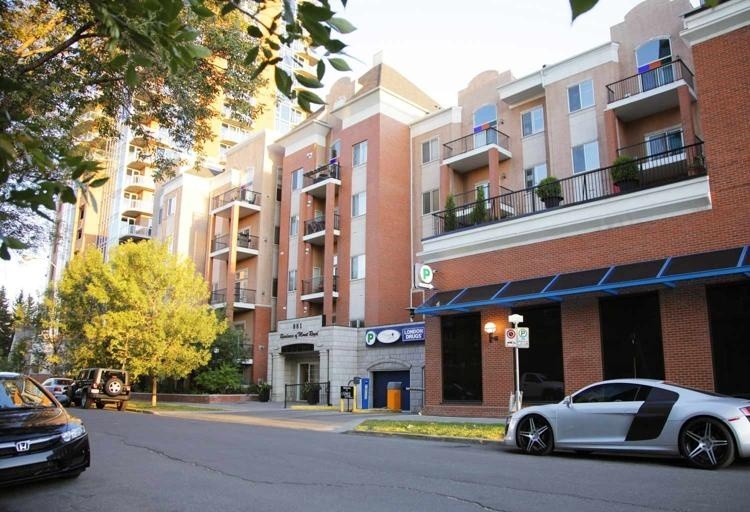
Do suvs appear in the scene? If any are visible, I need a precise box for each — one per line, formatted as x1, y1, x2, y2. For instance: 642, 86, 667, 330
69, 368, 131, 411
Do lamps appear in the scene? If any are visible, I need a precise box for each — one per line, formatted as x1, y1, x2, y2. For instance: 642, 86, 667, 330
484, 322, 498, 343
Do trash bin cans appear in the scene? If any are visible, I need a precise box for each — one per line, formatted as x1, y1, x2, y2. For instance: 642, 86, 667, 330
387, 382, 402, 413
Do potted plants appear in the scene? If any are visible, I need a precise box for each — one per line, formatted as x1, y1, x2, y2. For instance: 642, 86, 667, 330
303, 382, 322, 405
611, 155, 640, 192
534, 176, 564, 208
687, 153, 706, 176
255, 382, 272, 401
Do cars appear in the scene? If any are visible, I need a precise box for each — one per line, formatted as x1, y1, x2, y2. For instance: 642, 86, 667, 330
36, 378, 74, 407
0, 372, 91, 490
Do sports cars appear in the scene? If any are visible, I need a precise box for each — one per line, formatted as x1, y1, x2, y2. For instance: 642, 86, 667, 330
505, 378, 750, 470
